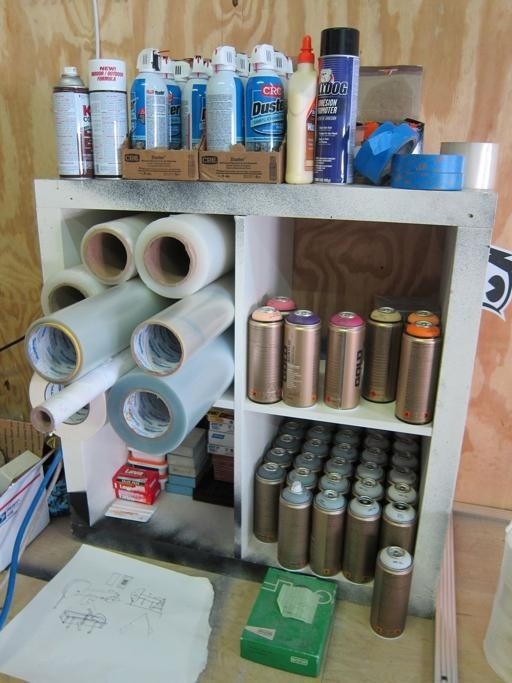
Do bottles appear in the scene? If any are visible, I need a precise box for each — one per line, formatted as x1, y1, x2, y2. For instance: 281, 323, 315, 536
369, 544, 411, 642
250, 294, 445, 428
87, 56, 130, 183
285, 33, 315, 185
130, 44, 294, 185
315, 26, 359, 186
56, 63, 94, 184
250, 419, 419, 587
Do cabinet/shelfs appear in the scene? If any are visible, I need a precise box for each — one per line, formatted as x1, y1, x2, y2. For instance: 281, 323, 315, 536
25, 176, 496, 620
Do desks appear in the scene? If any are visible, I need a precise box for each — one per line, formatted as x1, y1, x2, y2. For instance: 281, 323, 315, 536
2, 497, 510, 681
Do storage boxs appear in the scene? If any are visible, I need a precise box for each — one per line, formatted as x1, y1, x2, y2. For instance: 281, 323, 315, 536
120, 136, 199, 180
197, 141, 287, 182
1, 416, 50, 576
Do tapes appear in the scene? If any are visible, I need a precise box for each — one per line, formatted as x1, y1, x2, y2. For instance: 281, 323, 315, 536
354, 122, 499, 191
22, 204, 234, 457
